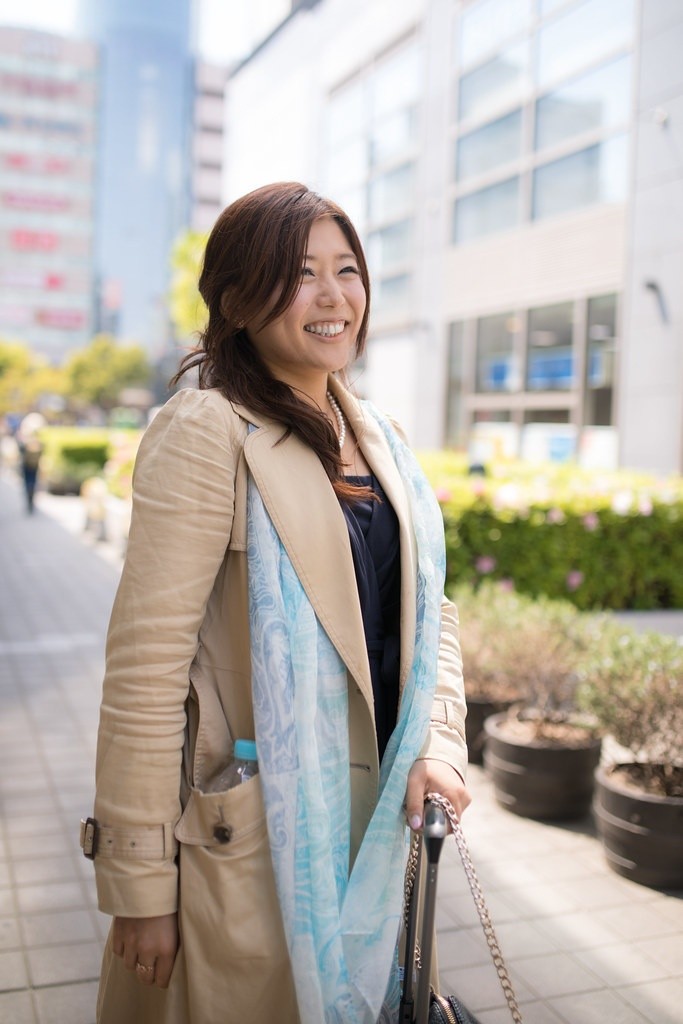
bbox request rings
[136,961,155,973]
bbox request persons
[19,430,45,512]
[77,183,472,1024]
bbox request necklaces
[326,391,351,449]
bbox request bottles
[206,739,259,794]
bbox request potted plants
[447,573,683,893]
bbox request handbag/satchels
[403,793,521,1024]
